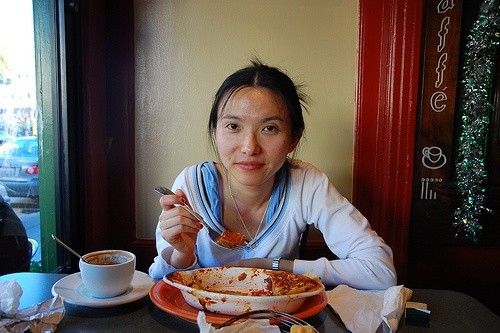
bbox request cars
[0,129,39,208]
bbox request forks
[153,185,245,253]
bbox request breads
[289,324,316,333]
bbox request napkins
[325,284,432,333]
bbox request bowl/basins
[163,265,326,316]
[79,250,138,299]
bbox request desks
[0,272,499,333]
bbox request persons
[0,195,32,276]
[150,48,397,291]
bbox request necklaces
[227,170,272,241]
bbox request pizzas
[218,230,245,247]
[270,271,318,296]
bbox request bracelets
[272,256,282,270]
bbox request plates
[51,271,154,307]
[150,278,328,327]
